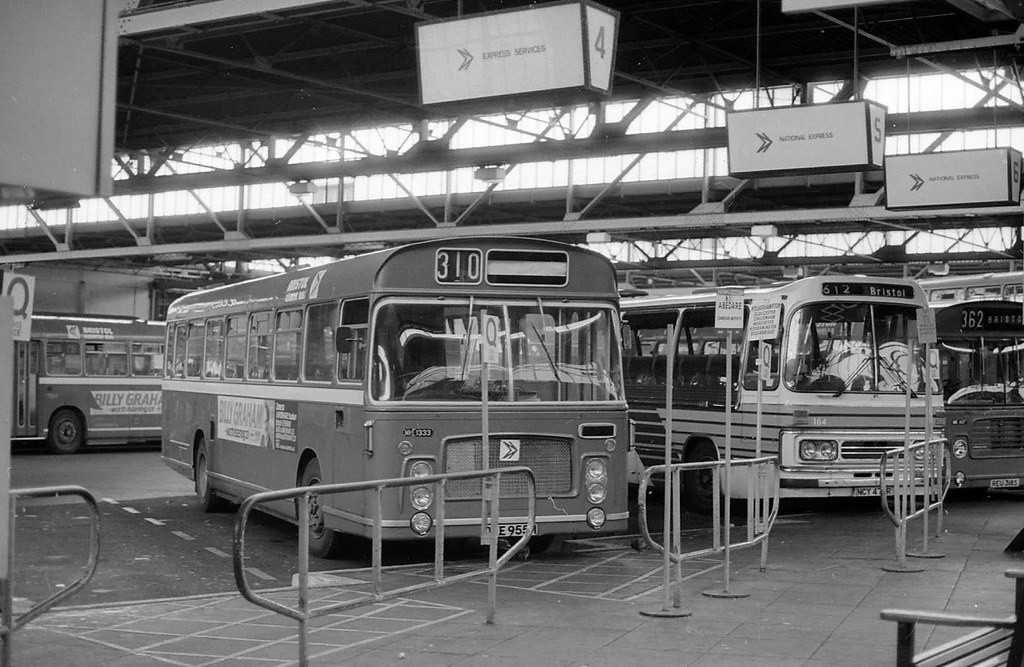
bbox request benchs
[881,570,1024,667]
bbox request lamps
[474,163,505,183]
[782,266,805,278]
[584,233,613,244]
[928,263,950,275]
[290,180,315,193]
[751,224,778,237]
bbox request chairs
[623,353,737,389]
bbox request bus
[160,235,633,560]
[852,298,1024,498]
[917,269,1024,303]
[13,315,174,453]
[611,273,946,513]
